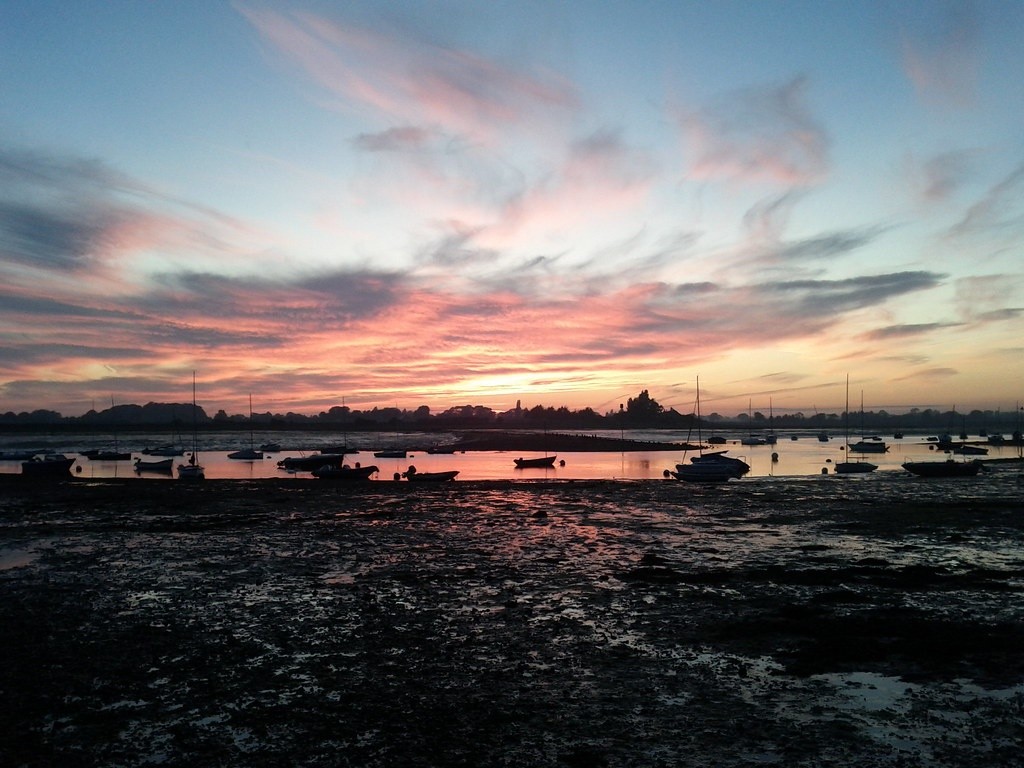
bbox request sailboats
[959,414,968,439]
[88,394,131,460]
[320,397,358,454]
[676,377,750,479]
[227,394,263,459]
[767,398,777,443]
[176,371,205,478]
[150,405,185,456]
[78,398,99,456]
[834,381,880,473]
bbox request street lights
[620,404,623,440]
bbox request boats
[311,466,379,479]
[791,435,798,440]
[954,445,989,455]
[987,435,1005,441]
[284,455,344,471]
[403,471,460,482]
[513,457,556,466]
[374,449,406,458]
[901,460,979,477]
[427,447,457,454]
[819,436,828,441]
[1012,431,1023,440]
[894,433,903,439]
[133,458,174,470]
[34,449,55,454]
[740,437,767,445]
[980,429,986,436]
[260,444,280,451]
[708,436,727,444]
[818,434,827,438]
[873,437,882,441]
[927,437,938,441]
[669,471,731,482]
[847,441,886,454]
